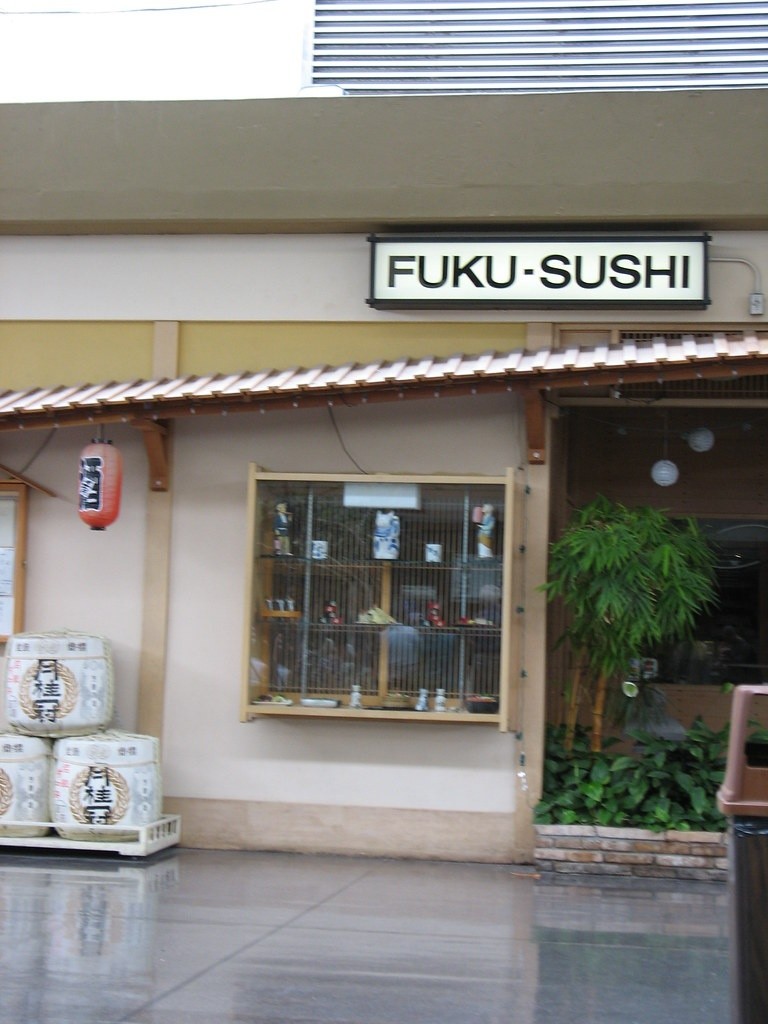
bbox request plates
[252,701,293,706]
[355,621,403,625]
[452,624,495,628]
[301,699,338,707]
[369,706,416,711]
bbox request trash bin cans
[716,685,768,1023]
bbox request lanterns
[76,439,123,531]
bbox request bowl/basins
[465,696,499,713]
[385,696,410,707]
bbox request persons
[273,504,294,555]
[378,621,425,696]
[477,504,496,558]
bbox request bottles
[435,689,446,712]
[416,689,428,711]
[349,685,362,710]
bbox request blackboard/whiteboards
[0,478,28,643]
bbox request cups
[312,541,328,560]
[426,544,442,562]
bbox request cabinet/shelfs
[244,463,522,733]
